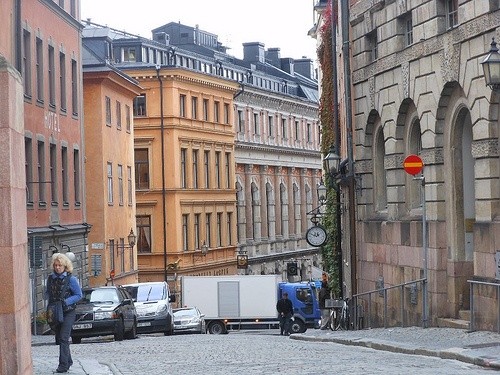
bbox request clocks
[307,226,326,247]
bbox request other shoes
[56,359,73,372]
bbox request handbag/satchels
[46,300,63,324]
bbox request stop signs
[404,153,424,176]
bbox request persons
[44,253,82,372]
[276,293,293,336]
[319,280,330,329]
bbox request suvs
[122,281,174,337]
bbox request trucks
[181,275,323,336]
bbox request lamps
[194,241,208,256]
[50,245,61,261]
[106,228,137,248]
[62,244,75,261]
[318,176,341,207]
[480,38,500,90]
[324,146,348,193]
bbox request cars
[172,307,207,335]
[70,284,138,344]
